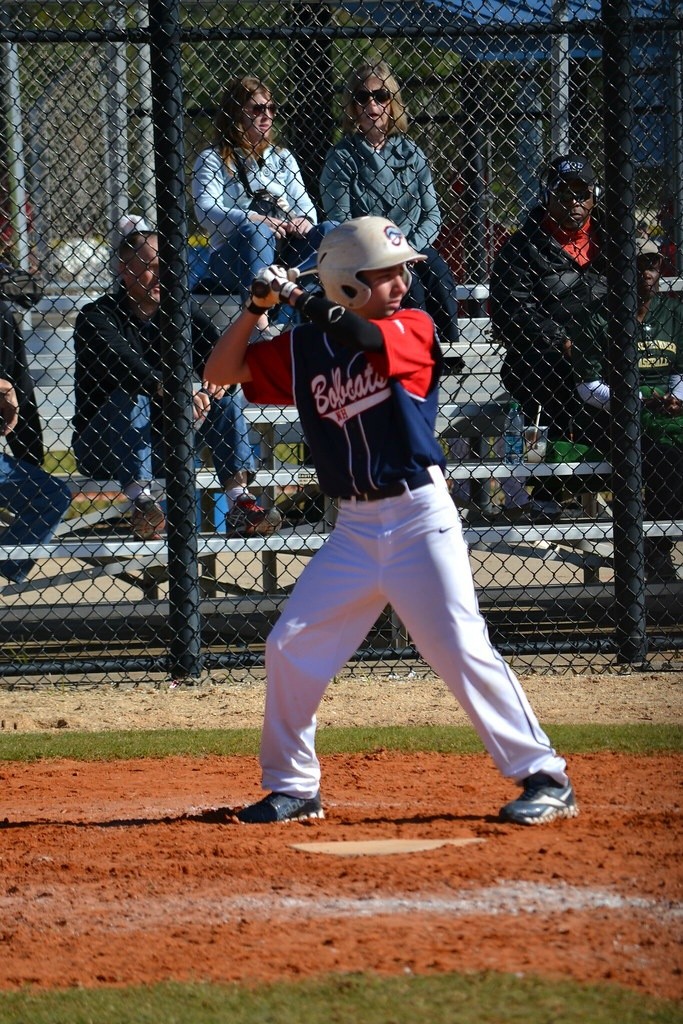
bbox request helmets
[317,215,428,310]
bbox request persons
[202,215,582,826]
[71,231,283,542]
[190,78,341,337]
[490,155,683,581]
[0,263,73,594]
[321,60,467,375]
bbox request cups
[522,426,550,462]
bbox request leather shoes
[339,469,434,501]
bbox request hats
[635,238,665,260]
[548,155,594,189]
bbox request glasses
[355,89,392,104]
[242,104,278,116]
[553,184,591,203]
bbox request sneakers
[499,772,580,826]
[232,791,325,824]
[131,496,166,541]
[225,492,282,537]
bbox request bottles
[504,401,524,465]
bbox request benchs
[0,276,683,646]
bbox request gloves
[244,263,300,316]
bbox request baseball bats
[252,268,319,299]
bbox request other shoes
[250,329,273,344]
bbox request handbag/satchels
[249,188,296,258]
